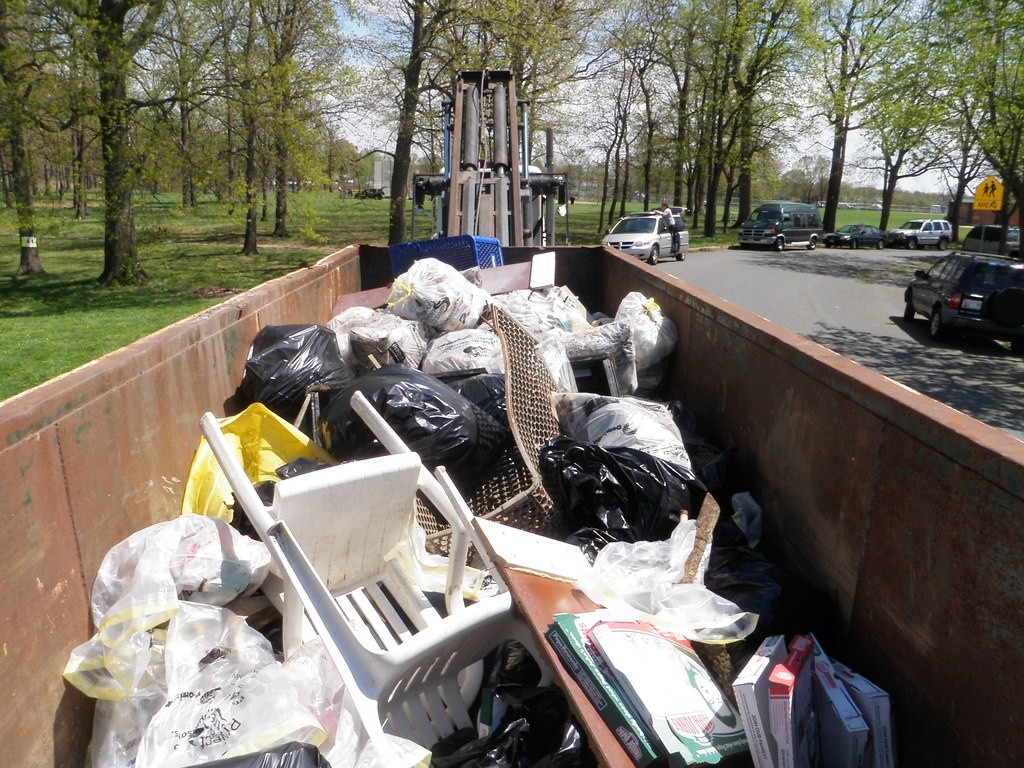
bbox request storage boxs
[546,605,893,768]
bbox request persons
[569,195,576,204]
[654,196,680,252]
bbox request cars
[822,223,894,249]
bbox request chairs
[201,304,570,768]
[968,271,1014,293]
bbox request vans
[737,201,824,251]
[602,207,690,266]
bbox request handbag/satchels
[62,516,272,702]
[133,600,328,768]
[574,519,759,645]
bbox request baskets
[389,235,504,278]
[413,300,569,564]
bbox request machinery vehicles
[406,68,573,246]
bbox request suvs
[886,219,954,251]
[904,250,1024,355]
[355,188,386,200]
[962,225,1021,258]
